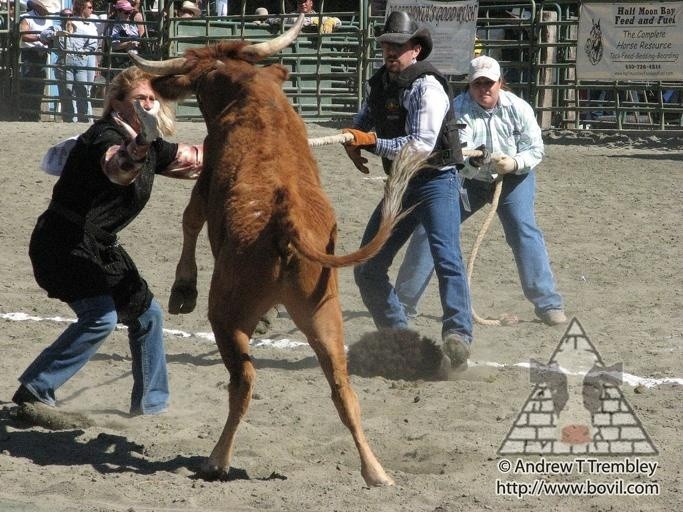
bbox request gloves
[469,144,491,166]
[133,98,161,141]
[495,154,516,175]
[345,144,370,175]
[340,128,378,149]
[311,18,336,36]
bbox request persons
[496,7,540,118]
[10,63,204,420]
[340,10,474,373]
[395,54,567,327]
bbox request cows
[126,12,438,488]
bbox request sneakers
[443,333,471,375]
[540,308,568,325]
[10,386,44,406]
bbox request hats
[469,55,501,82]
[112,0,135,11]
[372,11,433,62]
[31,0,73,16]
[505,8,531,21]
[179,0,202,16]
